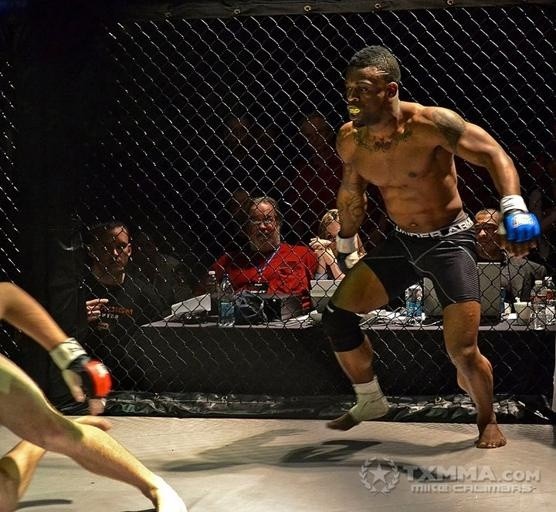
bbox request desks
[126,299,556,394]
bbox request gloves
[500,195,540,244]
[335,233,359,275]
[51,338,112,404]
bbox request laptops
[421,261,502,323]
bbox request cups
[513,302,534,320]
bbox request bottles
[206,270,220,316]
[217,272,236,328]
[544,276,556,327]
[530,280,547,331]
[404,282,423,327]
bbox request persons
[319,46,542,449]
[83,220,172,392]
[0,281,186,512]
[192,115,555,313]
